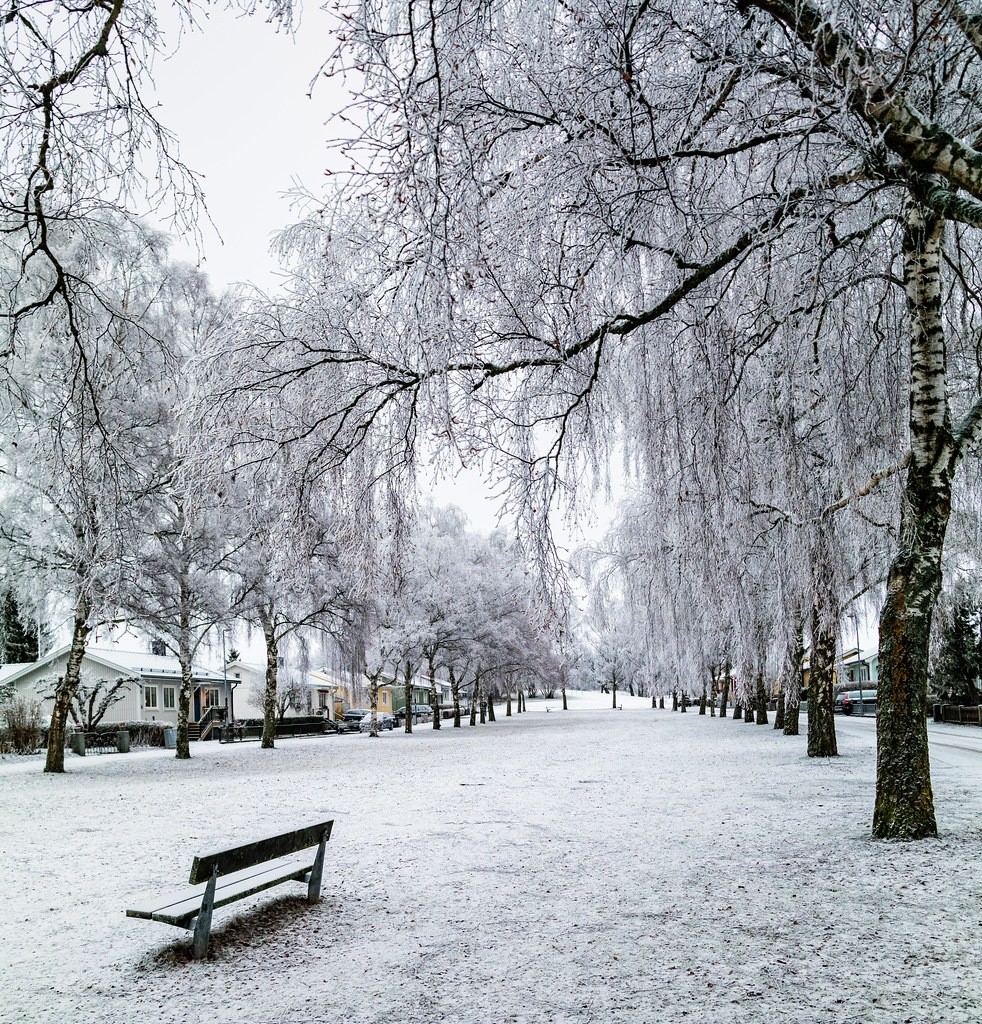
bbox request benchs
[125,819,334,959]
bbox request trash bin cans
[164,729,177,749]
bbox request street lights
[222,629,232,743]
[847,613,864,717]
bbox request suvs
[835,689,878,714]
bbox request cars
[393,704,434,720]
[337,708,394,734]
